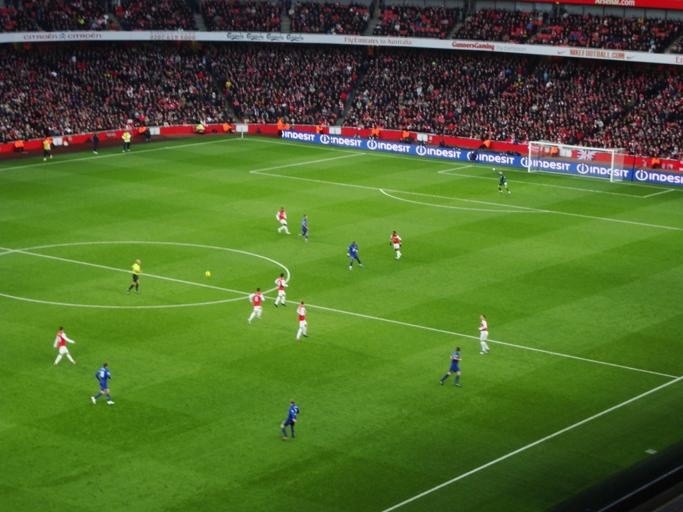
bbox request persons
[299,213,310,241]
[126,259,142,294]
[276,207,291,235]
[390,229,403,259]
[296,301,308,340]
[90,362,116,406]
[439,346,464,388]
[274,273,289,308]
[347,240,363,271]
[279,400,299,440]
[478,314,490,355]
[248,288,266,325]
[52,327,77,366]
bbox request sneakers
[91,397,115,406]
[347,253,403,272]
[498,190,511,195]
[282,435,297,440]
[127,290,141,296]
[246,301,285,326]
[53,360,78,367]
[296,334,307,341]
[480,348,490,355]
[439,379,462,390]
[277,228,309,242]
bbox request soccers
[205,271,210,276]
